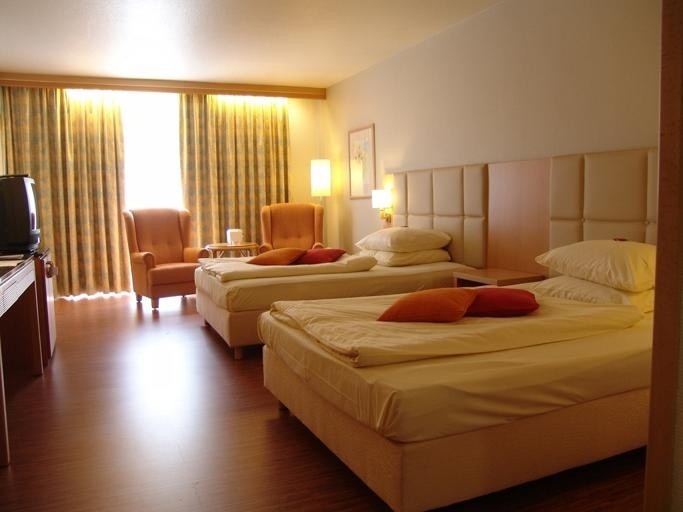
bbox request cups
[227,229,242,245]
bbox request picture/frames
[348,123,376,198]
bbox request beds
[257,284,655,512]
[194,253,475,363]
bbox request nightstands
[450,267,545,287]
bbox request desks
[204,241,255,258]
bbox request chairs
[123,207,203,310]
[259,199,324,253]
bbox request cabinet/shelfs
[0,249,59,467]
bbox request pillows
[250,248,305,264]
[470,289,538,317]
[354,227,452,250]
[300,247,346,263]
[534,237,656,291]
[377,286,475,320]
[366,251,452,267]
[528,276,655,307]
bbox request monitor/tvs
[0,176,42,254]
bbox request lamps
[310,160,332,205]
[371,190,392,225]
